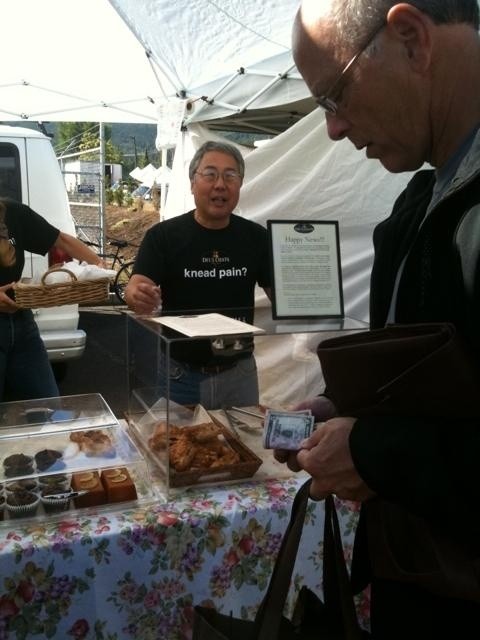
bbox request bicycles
[80,233,137,306]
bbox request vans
[76,182,95,198]
[0,124,77,284]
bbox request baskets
[13,263,112,310]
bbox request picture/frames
[267,218,344,319]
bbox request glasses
[313,22,385,117]
[195,169,243,184]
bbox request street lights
[130,135,137,169]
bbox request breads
[0,430,137,521]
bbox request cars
[110,181,161,208]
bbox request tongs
[222,403,266,438]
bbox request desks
[0,406,372,640]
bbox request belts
[171,354,252,377]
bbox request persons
[122,140,273,412]
[1,201,106,426]
[272,0,478,638]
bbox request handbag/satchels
[189,479,379,638]
[315,320,479,598]
